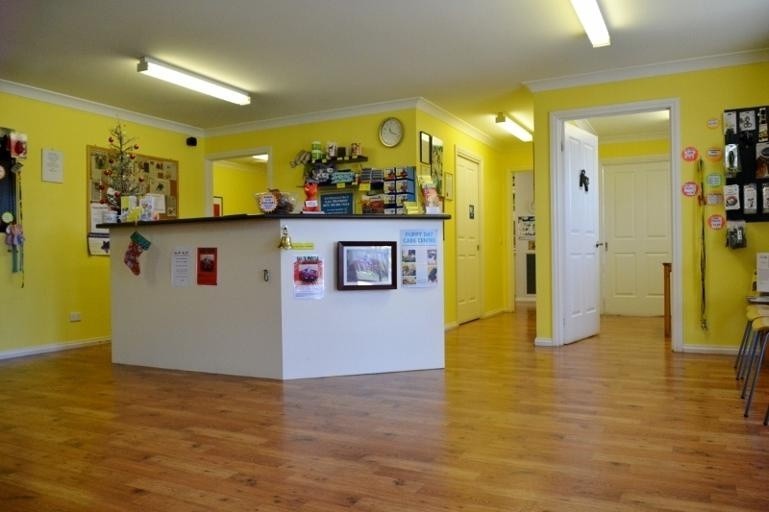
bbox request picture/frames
[419,131,432,166]
[336,240,398,292]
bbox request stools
[732,296,769,426]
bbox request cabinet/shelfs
[295,155,383,190]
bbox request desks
[92,211,450,382]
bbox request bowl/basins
[254,192,299,214]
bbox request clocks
[377,115,403,148]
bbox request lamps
[136,55,252,106]
[496,112,533,144]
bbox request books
[416,174,441,214]
